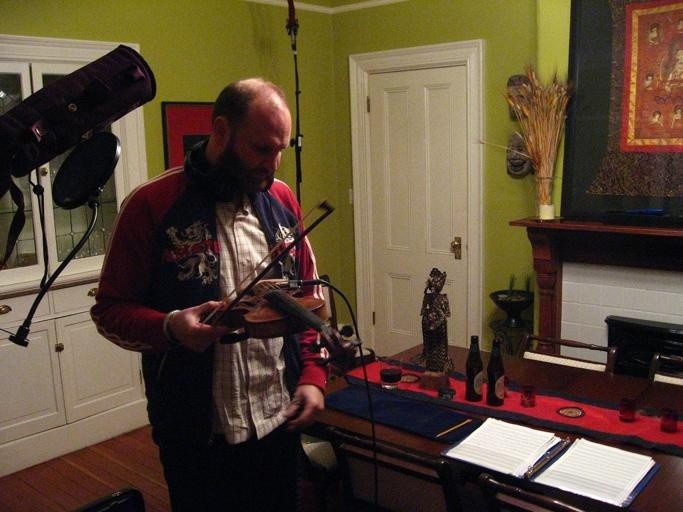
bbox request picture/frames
[162,102,215,169]
[561,0,683,227]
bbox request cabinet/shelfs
[1,34,147,475]
[509,218,683,432]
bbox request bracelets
[164,310,181,348]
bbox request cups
[378,354,403,390]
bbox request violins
[88,201,362,370]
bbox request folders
[441,417,659,507]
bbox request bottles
[486,339,504,406]
[465,335,482,402]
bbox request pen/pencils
[436,419,472,437]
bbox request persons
[91,77,332,511]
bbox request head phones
[183,137,275,203]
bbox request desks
[296,342,683,512]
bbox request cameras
[1,44,158,348]
